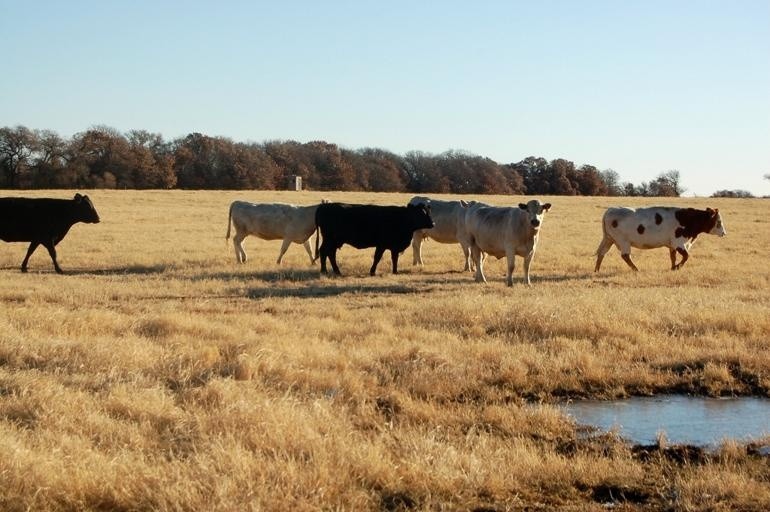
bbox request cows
[224,199,332,267]
[463,199,553,287]
[407,195,482,274]
[312,200,435,275]
[589,205,730,274]
[0,192,102,274]
